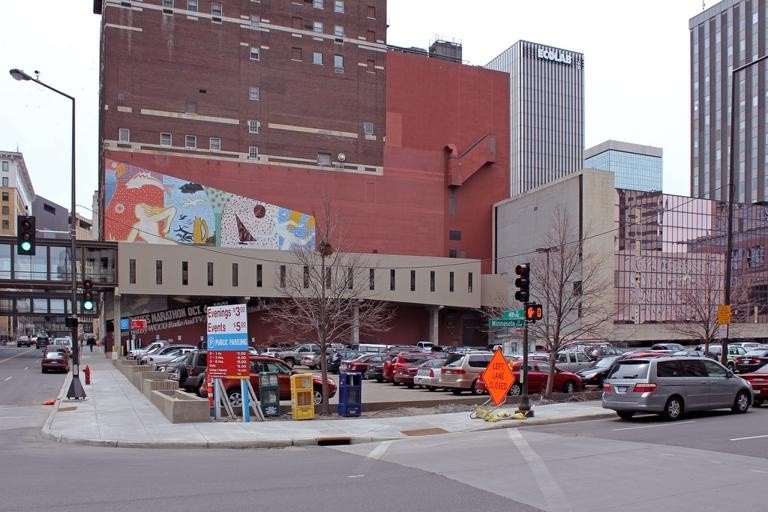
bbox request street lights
[9,69,86,401]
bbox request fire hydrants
[81,364,90,385]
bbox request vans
[601,356,753,421]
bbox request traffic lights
[515,263,529,301]
[16,214,35,254]
[526,304,543,320]
[84,280,94,312]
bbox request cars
[41,352,69,373]
[16,335,73,357]
[43,344,68,353]
[695,341,768,374]
[622,352,671,359]
[651,344,685,352]
[198,355,336,408]
[672,350,719,364]
[739,364,768,408]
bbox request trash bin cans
[289,372,316,421]
[259,371,281,417]
[338,370,363,418]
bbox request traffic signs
[489,320,524,329]
[503,309,524,319]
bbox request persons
[87,335,97,351]
